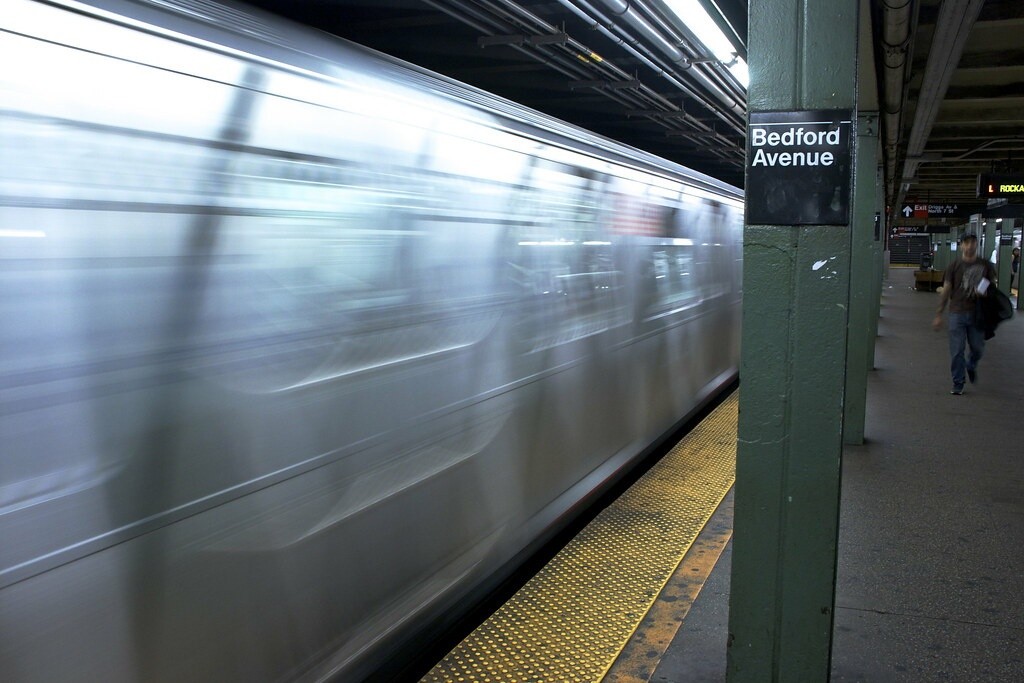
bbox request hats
[960,232,977,242]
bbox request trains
[0,2,747,682]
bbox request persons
[1010,247,1019,297]
[932,233,996,396]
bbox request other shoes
[1009,293,1015,296]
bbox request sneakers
[950,383,965,395]
[967,366,978,385]
